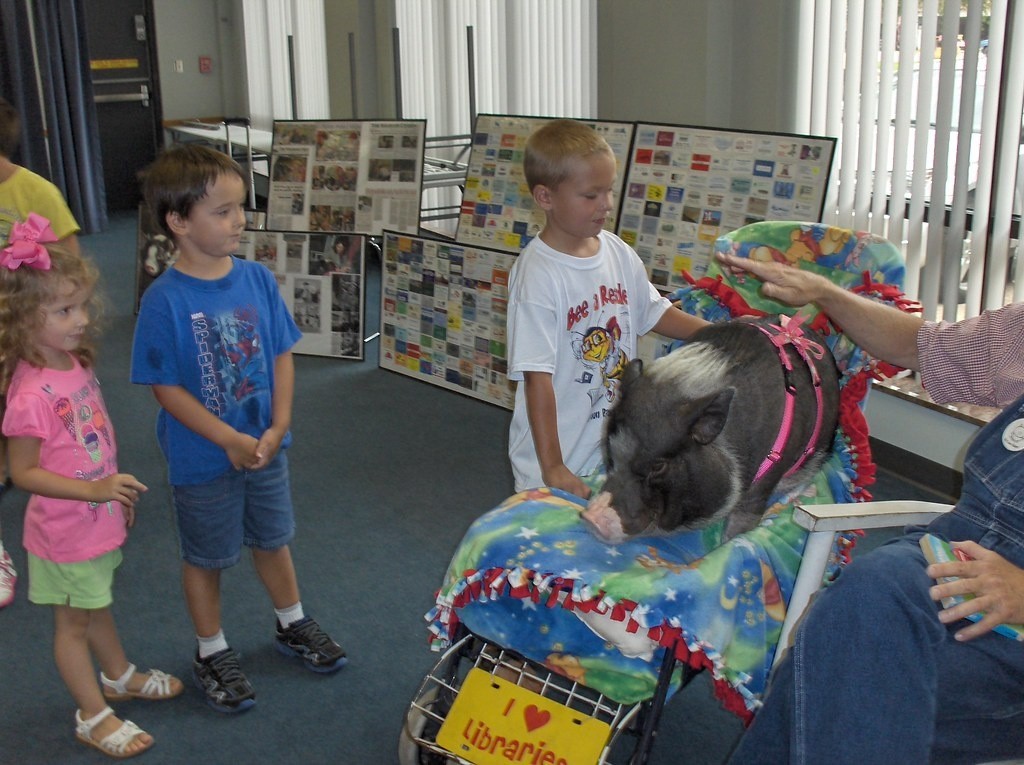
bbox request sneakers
[191,640,257,716]
[0,550,18,606]
[275,611,348,671]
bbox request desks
[167,25,481,223]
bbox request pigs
[580,313,841,544]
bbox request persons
[0,213,185,757]
[716,251,1024,765]
[130,143,350,712]
[331,235,352,273]
[506,119,763,499]
[280,126,305,146]
[0,96,80,606]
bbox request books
[919,533,1024,641]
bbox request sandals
[75,704,155,758]
[100,661,184,701]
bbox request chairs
[770,482,954,693]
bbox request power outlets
[174,60,184,74]
